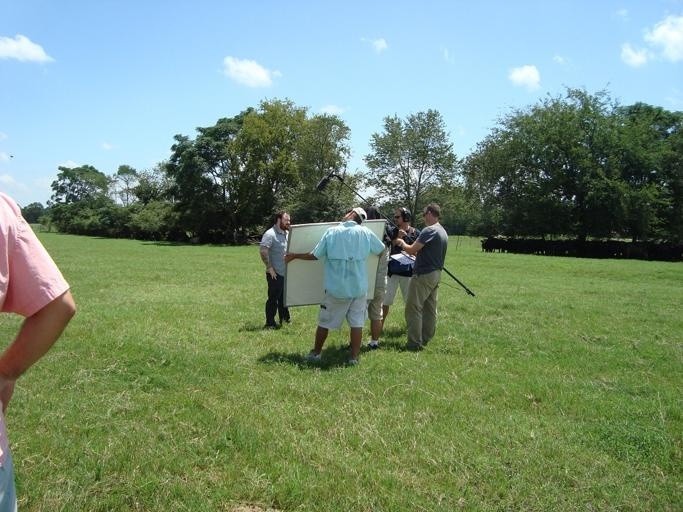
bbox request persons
[258,208,292,330]
[359,203,393,351]
[282,206,386,365]
[381,207,421,333]
[0,191,76,512]
[391,203,448,352]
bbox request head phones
[402,207,410,222]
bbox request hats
[353,207,367,223]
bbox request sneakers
[366,344,380,350]
[308,350,324,361]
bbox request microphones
[317,176,331,191]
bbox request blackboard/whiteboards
[281,218,389,309]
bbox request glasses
[395,215,400,219]
